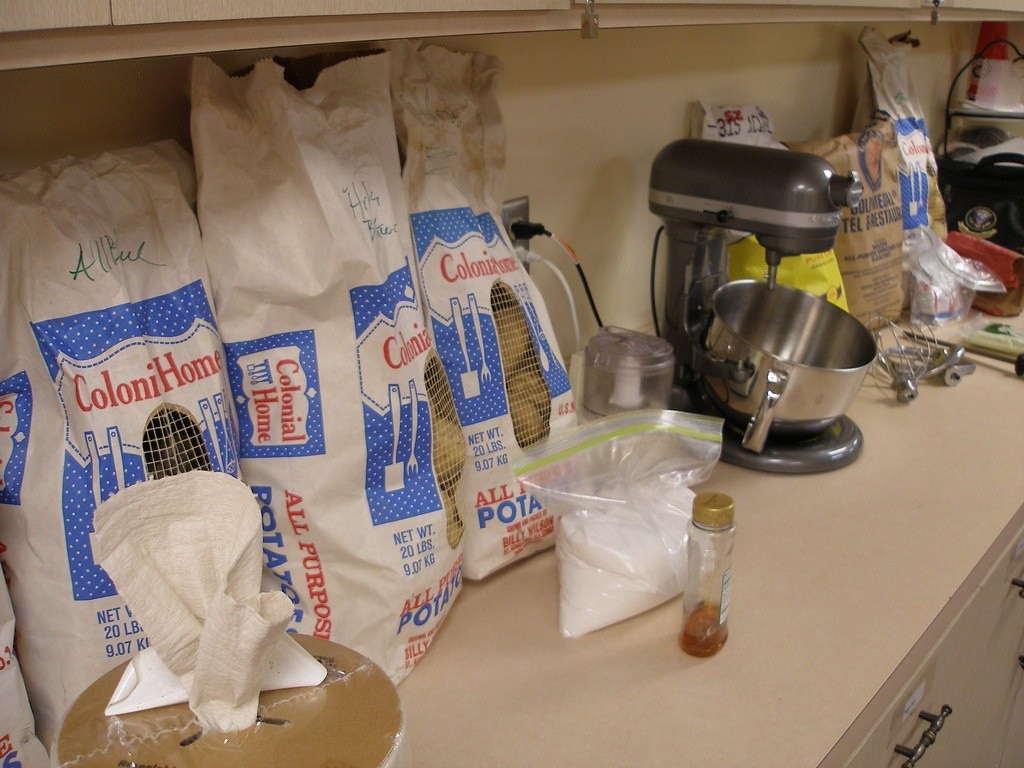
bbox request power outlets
[502,193,532,277]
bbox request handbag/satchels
[935,152,1024,258]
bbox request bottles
[679,491,737,656]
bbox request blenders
[647,137,882,476]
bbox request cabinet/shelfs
[833,534,1024,767]
